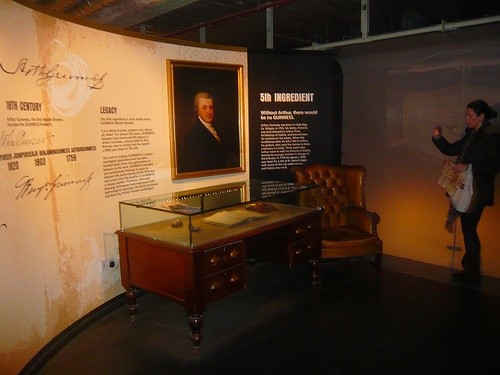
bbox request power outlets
[102,258,116,272]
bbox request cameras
[434,129,440,138]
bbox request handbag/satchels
[451,164,473,213]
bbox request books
[201,208,269,228]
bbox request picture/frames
[166,59,247,180]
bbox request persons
[187,93,225,173]
[432,100,500,286]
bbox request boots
[452,254,480,285]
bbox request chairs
[292,163,383,272]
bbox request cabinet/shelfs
[116,201,324,354]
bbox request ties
[210,127,222,142]
